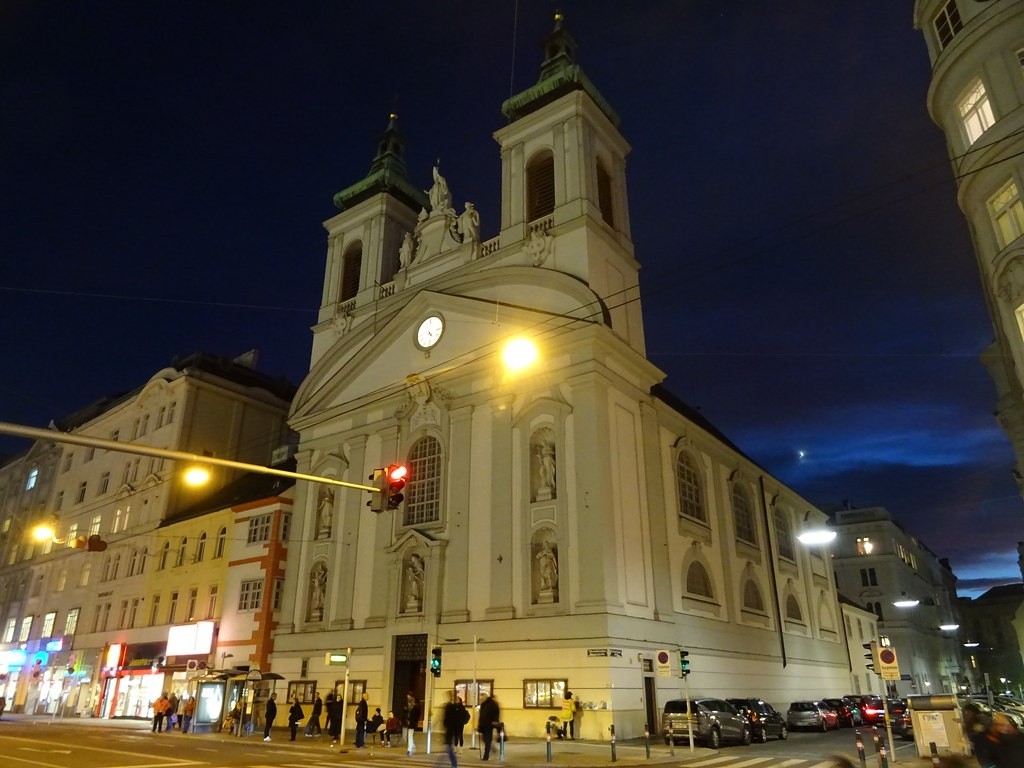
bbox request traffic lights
[68,655,78,674]
[30,659,42,679]
[388,464,408,511]
[862,643,874,671]
[431,642,444,677]
[679,650,690,681]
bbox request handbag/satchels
[171,714,178,723]
[464,710,471,724]
[227,711,233,718]
[164,708,173,716]
[496,721,508,743]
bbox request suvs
[822,697,864,728]
[726,697,788,744]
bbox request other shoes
[151,729,155,733]
[483,757,488,761]
[264,736,273,742]
[313,733,322,738]
[333,739,337,744]
[359,745,367,749]
[157,730,163,733]
[412,746,416,753]
[459,747,464,754]
[288,738,296,742]
[305,733,313,737]
[381,741,384,746]
[569,737,575,740]
[562,736,568,740]
[228,732,230,735]
[453,745,458,753]
[407,751,412,756]
[182,731,187,734]
[384,742,391,748]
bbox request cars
[788,700,841,734]
[661,697,752,749]
[844,693,1024,746]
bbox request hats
[162,692,169,700]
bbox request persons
[263,692,277,742]
[456,202,481,242]
[305,691,322,738]
[537,441,556,488]
[535,540,558,590]
[424,167,448,209]
[477,695,500,760]
[407,555,424,600]
[439,691,471,768]
[354,693,383,750]
[223,696,255,737]
[561,692,576,740]
[311,564,327,609]
[402,692,422,756]
[149,692,196,734]
[288,698,304,741]
[380,711,401,748]
[325,690,343,744]
[399,231,414,269]
[320,486,332,529]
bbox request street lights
[470,633,486,752]
[219,651,234,671]
